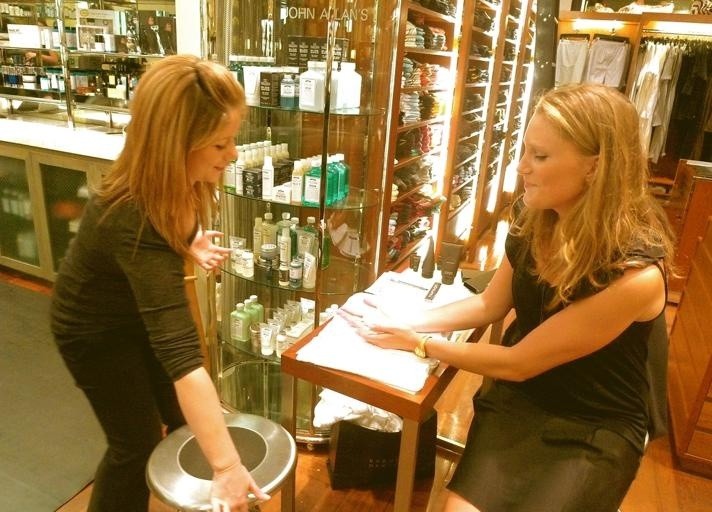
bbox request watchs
[414,336,432,360]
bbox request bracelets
[212,459,241,477]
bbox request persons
[356,81,680,511]
[46,49,273,512]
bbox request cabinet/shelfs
[665,158,712,479]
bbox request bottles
[223,139,352,358]
[0,0,137,101]
[1,189,32,219]
[279,59,363,116]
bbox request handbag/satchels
[326,408,437,489]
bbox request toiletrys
[222,294,349,359]
[217,210,339,290]
[216,137,352,207]
[218,58,366,118]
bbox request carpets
[1,278,110,510]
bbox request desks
[281,269,505,511]
